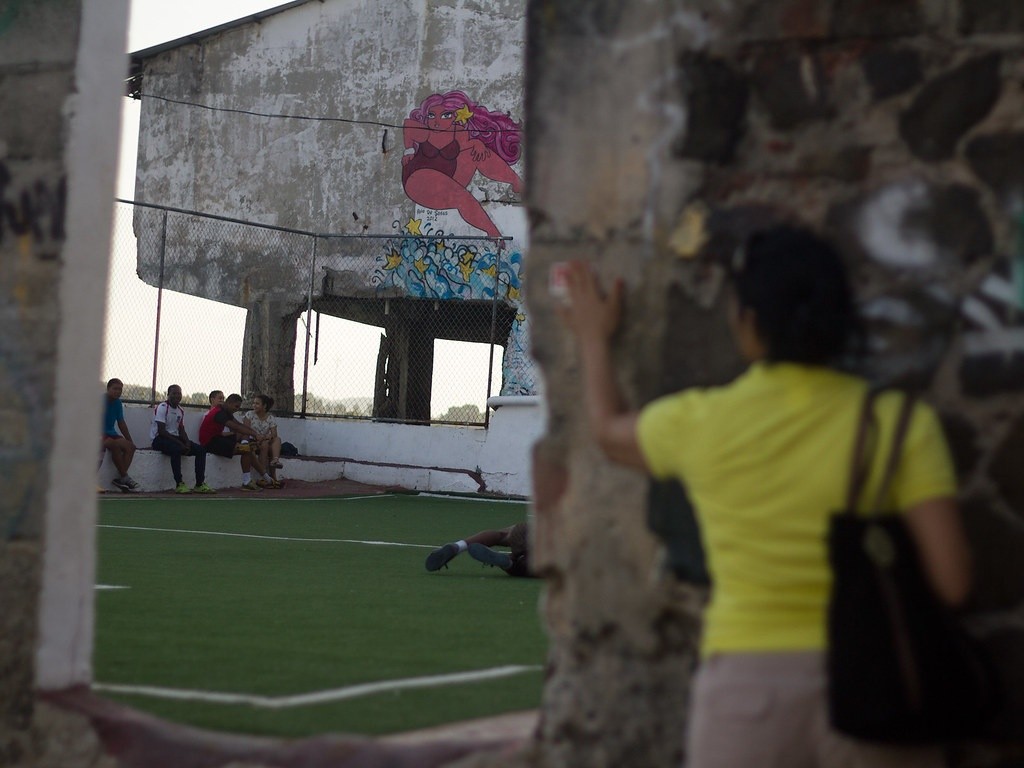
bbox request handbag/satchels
[280,442,299,457]
[824,378,1024,750]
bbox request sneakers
[240,479,263,492]
[112,477,143,492]
[193,481,216,494]
[175,482,192,494]
[236,442,261,453]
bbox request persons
[199,389,283,492]
[549,221,984,768]
[424,521,528,576]
[150,384,216,493]
[101,377,141,492]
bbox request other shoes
[466,542,512,570]
[426,542,458,572]
[271,479,281,489]
[96,484,109,493]
[257,480,274,489]
[269,458,283,469]
